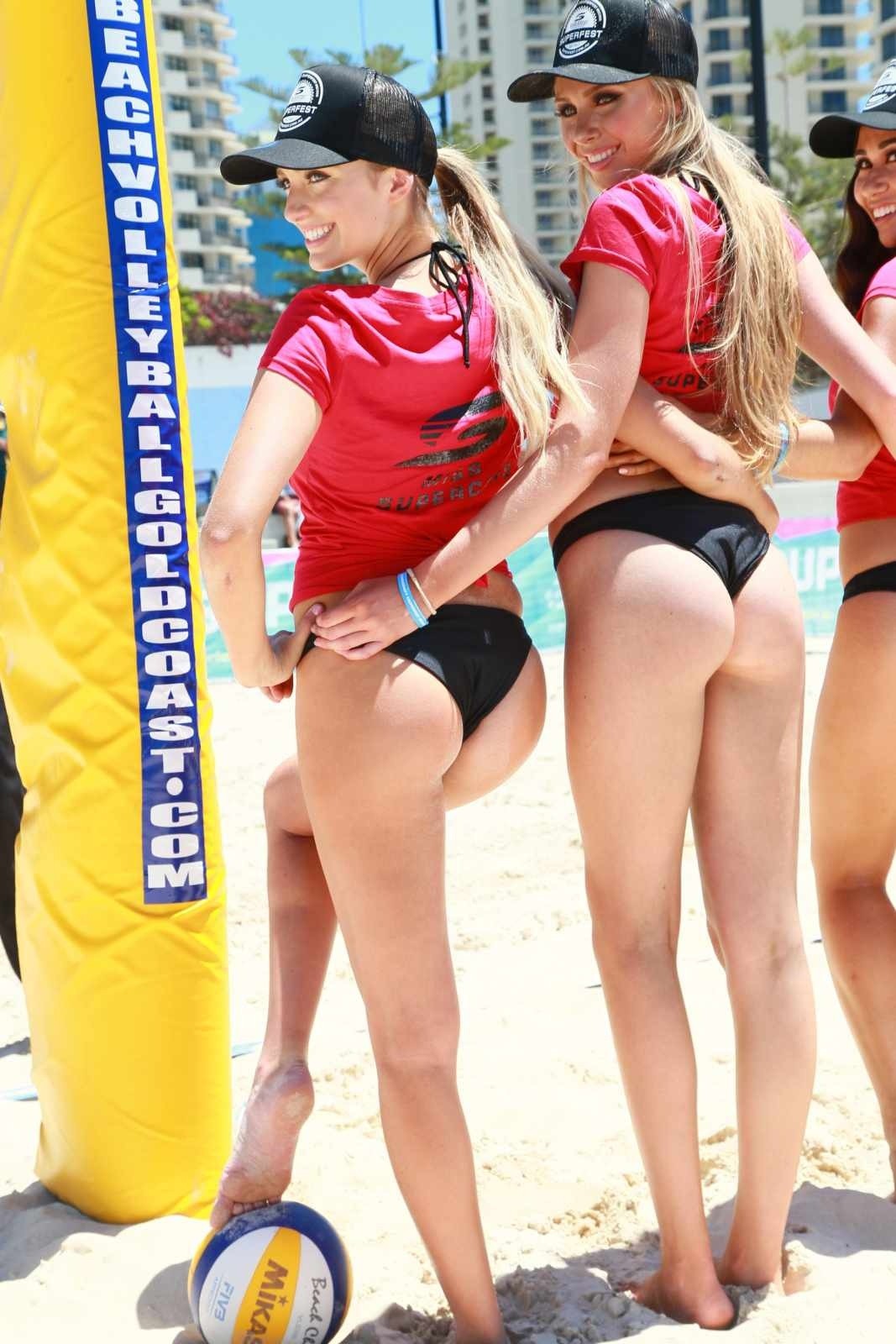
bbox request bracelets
[772,417,791,476]
[396,571,429,628]
[406,567,437,616]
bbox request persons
[173,0,896,1344]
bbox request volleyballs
[188,1201,354,1344]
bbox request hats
[506,1,698,102]
[809,60,895,159]
[220,66,437,186]
